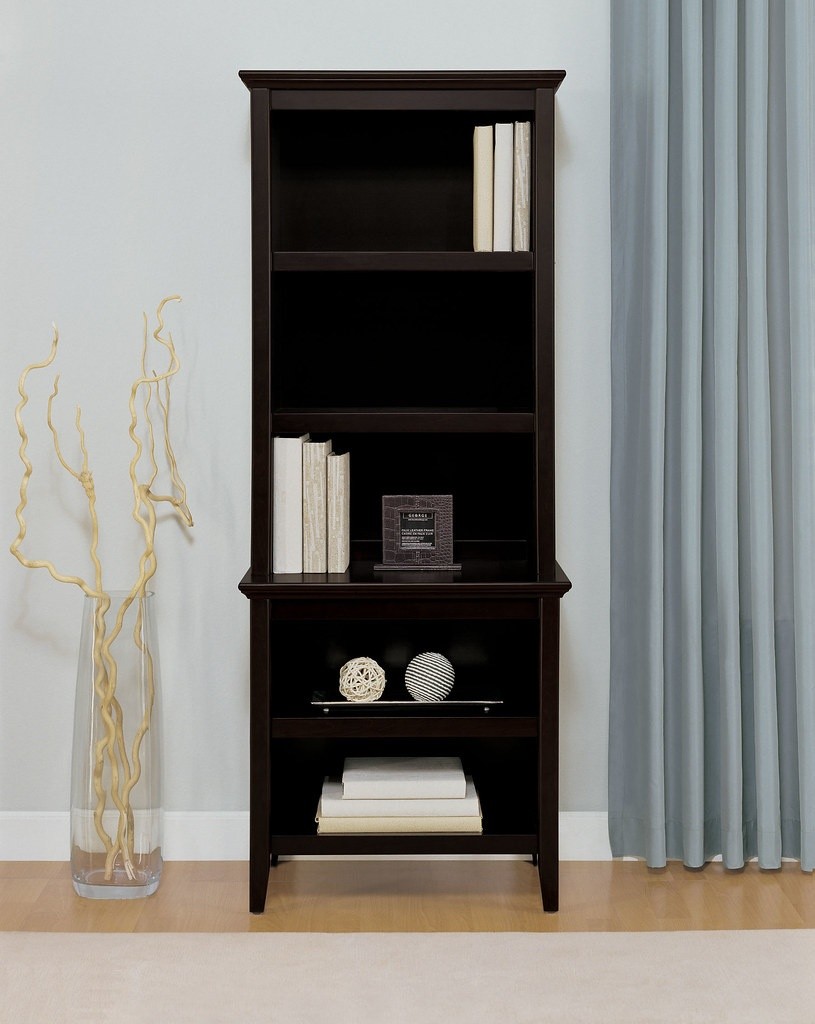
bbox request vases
[70,588,163,899]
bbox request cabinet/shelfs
[238,68,572,914]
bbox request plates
[310,689,504,707]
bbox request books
[472,122,533,253]
[317,755,483,834]
[273,434,349,574]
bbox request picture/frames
[381,494,454,566]
